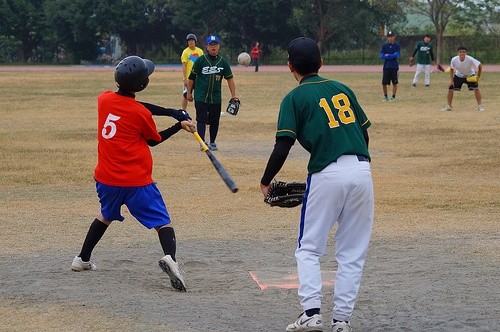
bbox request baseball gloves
[226,98,240,115]
[263,179,306,209]
[408,58,415,67]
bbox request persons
[250,42,262,73]
[260,37,374,332]
[380,31,400,103]
[180,34,203,110]
[441,46,485,113]
[411,35,436,87]
[186,36,238,152]
[69,55,196,291]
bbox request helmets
[114,56,155,92]
[186,33,197,44]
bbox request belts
[332,154,368,162]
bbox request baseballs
[238,52,251,65]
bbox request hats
[205,35,220,45]
[387,31,395,37]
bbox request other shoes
[478,104,484,111]
[441,105,452,112]
[382,96,389,102]
[389,97,395,101]
[200,142,218,151]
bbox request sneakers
[71,256,97,271]
[158,255,187,292]
[332,318,352,332]
[285,311,322,332]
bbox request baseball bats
[182,109,238,194]
